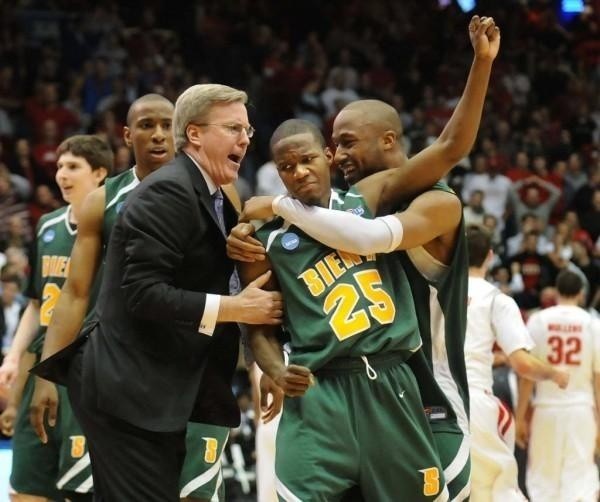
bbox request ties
[214,188,256,373]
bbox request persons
[2,135,111,501]
[235,15,506,501]
[0,0,205,347]
[456,223,567,498]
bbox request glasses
[190,122,255,138]
